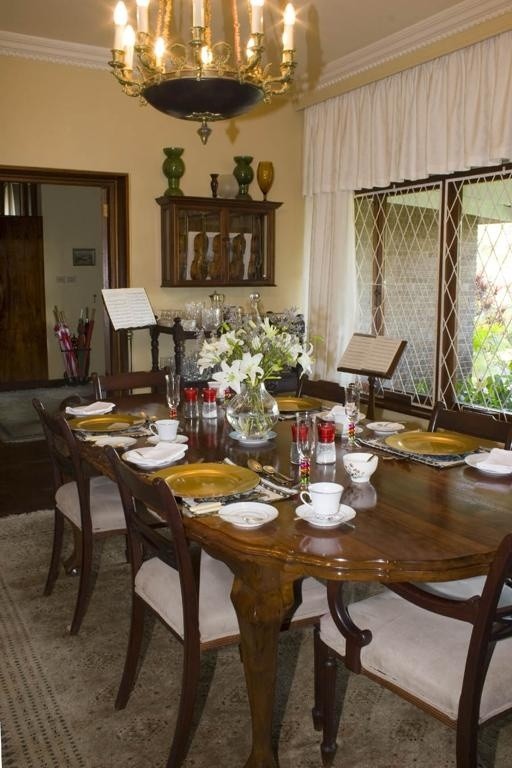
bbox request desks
[149,310,306,395]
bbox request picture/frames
[72,246,96,266]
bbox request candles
[125,46,133,67]
[193,0,204,27]
[282,24,294,50]
[251,4,265,33]
[136,5,150,34]
[113,25,124,50]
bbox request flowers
[197,317,322,392]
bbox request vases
[224,380,281,440]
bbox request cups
[160,304,246,329]
[300,483,344,515]
[150,420,179,440]
[163,355,213,380]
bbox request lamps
[108,0,296,144]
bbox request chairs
[31,394,169,636]
[316,530,512,767]
[428,399,512,448]
[90,364,173,400]
[296,373,357,405]
[102,442,330,766]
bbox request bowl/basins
[343,452,377,482]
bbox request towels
[66,401,115,415]
[326,404,346,420]
[130,441,189,464]
[478,448,512,474]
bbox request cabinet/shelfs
[155,194,283,287]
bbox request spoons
[247,459,287,484]
[263,465,293,482]
[210,512,262,521]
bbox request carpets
[1,382,107,442]
[2,507,498,764]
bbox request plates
[67,407,111,415]
[147,436,188,443]
[385,434,477,454]
[122,448,185,467]
[466,452,512,474]
[96,438,138,448]
[274,397,322,411]
[221,502,279,528]
[367,422,406,431]
[296,504,355,527]
[69,416,146,433]
[146,465,260,498]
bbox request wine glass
[166,375,180,419]
[345,381,360,446]
[296,413,317,492]
